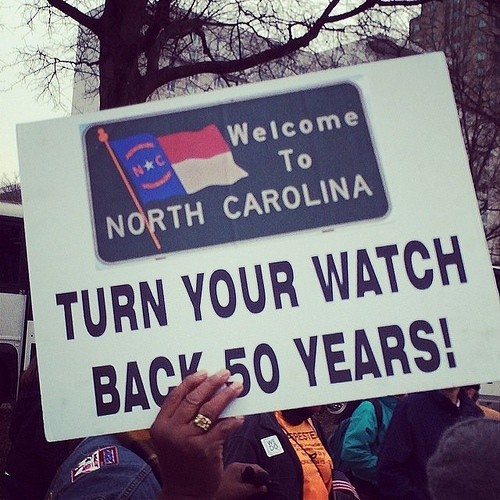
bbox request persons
[0,355,500,500]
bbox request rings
[194,413,212,431]
